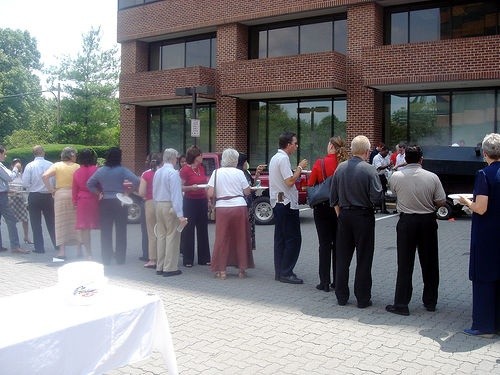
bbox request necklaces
[192,168,201,176]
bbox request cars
[126,174,142,224]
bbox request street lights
[172,86,214,144]
[296,106,329,152]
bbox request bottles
[177,218,188,232]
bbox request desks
[0,284,179,375]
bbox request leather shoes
[424,304,439,313]
[385,305,410,316]
[275,273,303,284]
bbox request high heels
[317,281,330,292]
[24,238,34,244]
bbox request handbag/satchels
[210,208,217,220]
[307,158,332,205]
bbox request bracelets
[298,166,303,170]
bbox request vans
[384,145,488,221]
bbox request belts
[343,206,367,209]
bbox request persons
[269,132,310,284]
[131,153,163,268]
[207,147,264,279]
[85,147,141,266]
[370,141,407,213]
[178,146,211,267]
[458,132,500,335]
[328,135,383,308]
[73,149,102,261]
[385,145,446,315]
[0,146,34,254]
[41,147,84,258]
[152,148,186,277]
[307,135,351,292]
[21,146,59,254]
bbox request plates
[250,187,268,189]
[116,193,133,204]
[448,194,473,200]
[197,184,208,187]
[154,224,157,237]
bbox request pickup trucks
[179,152,312,226]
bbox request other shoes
[12,247,29,254]
[359,299,372,309]
[144,261,157,268]
[56,255,64,259]
[239,271,247,279]
[215,271,227,280]
[205,261,210,264]
[463,328,496,338]
[381,210,389,214]
[139,256,148,262]
[32,249,38,254]
[156,271,163,274]
[163,270,181,277]
[186,263,192,267]
[337,300,346,304]
[0,247,7,252]
